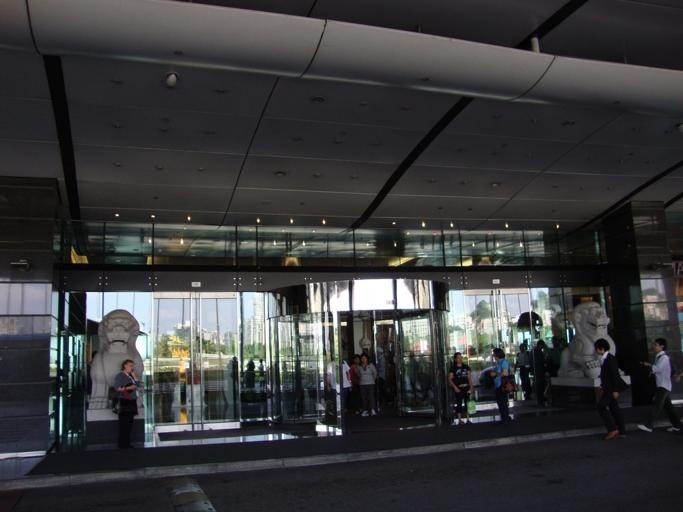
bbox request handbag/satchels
[500,374,519,394]
[466,400,478,416]
[108,386,136,401]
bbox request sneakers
[666,427,680,432]
[636,424,654,432]
[459,420,473,425]
[354,408,384,417]
[604,430,626,442]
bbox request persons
[447,351,473,426]
[404,350,433,406]
[489,347,513,426]
[227,356,266,390]
[85,350,97,412]
[114,359,139,448]
[594,338,628,443]
[327,345,387,417]
[514,335,566,401]
[637,338,682,433]
